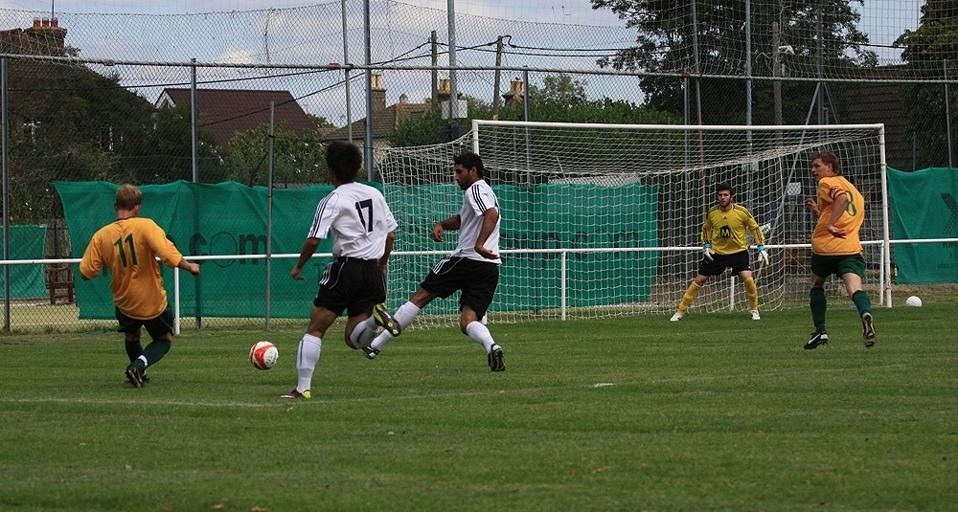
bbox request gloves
[758,246,769,266]
[703,244,716,264]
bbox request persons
[277,139,399,402]
[801,150,877,353]
[360,150,505,373]
[78,182,201,389]
[668,182,771,322]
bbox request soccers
[906,295,922,306]
[249,340,279,369]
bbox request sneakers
[804,330,829,350]
[488,344,507,372]
[862,312,876,348]
[280,387,311,399]
[373,303,401,337]
[670,313,683,321]
[750,309,760,320]
[125,364,150,389]
[362,344,380,360]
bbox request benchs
[45,258,75,305]
